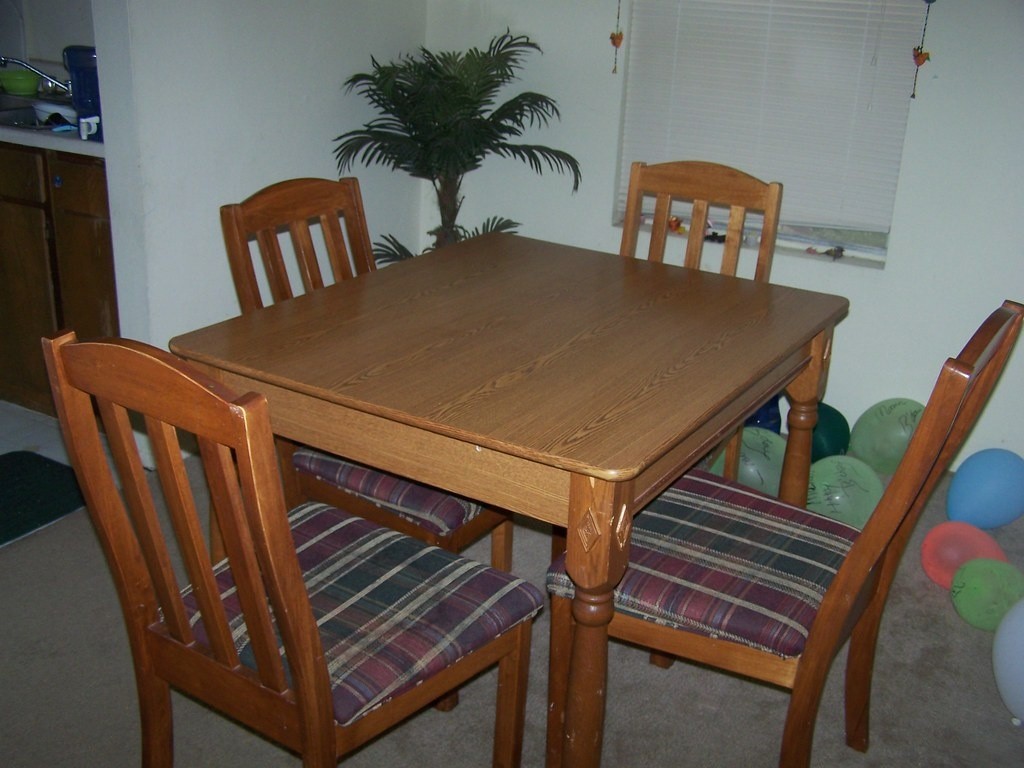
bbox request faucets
[0,56,73,98]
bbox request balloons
[916,448,1024,723]
[706,393,927,532]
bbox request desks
[170,232,847,766]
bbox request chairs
[218,177,516,575]
[621,160,782,480]
[543,301,1020,768]
[40,332,544,768]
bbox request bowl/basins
[0,68,42,94]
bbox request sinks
[0,103,49,132]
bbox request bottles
[64,45,101,128]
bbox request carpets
[0,452,86,546]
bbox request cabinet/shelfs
[1,143,129,427]
